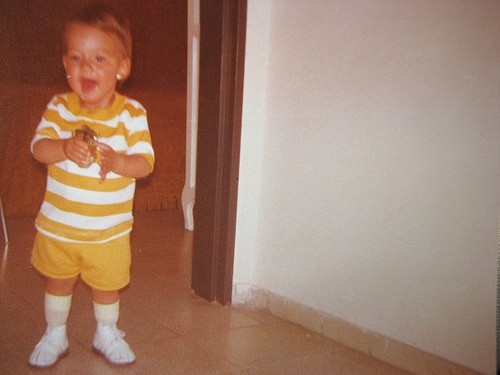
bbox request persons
[28,10,156,369]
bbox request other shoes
[92,322,137,367]
[30,330,70,367]
